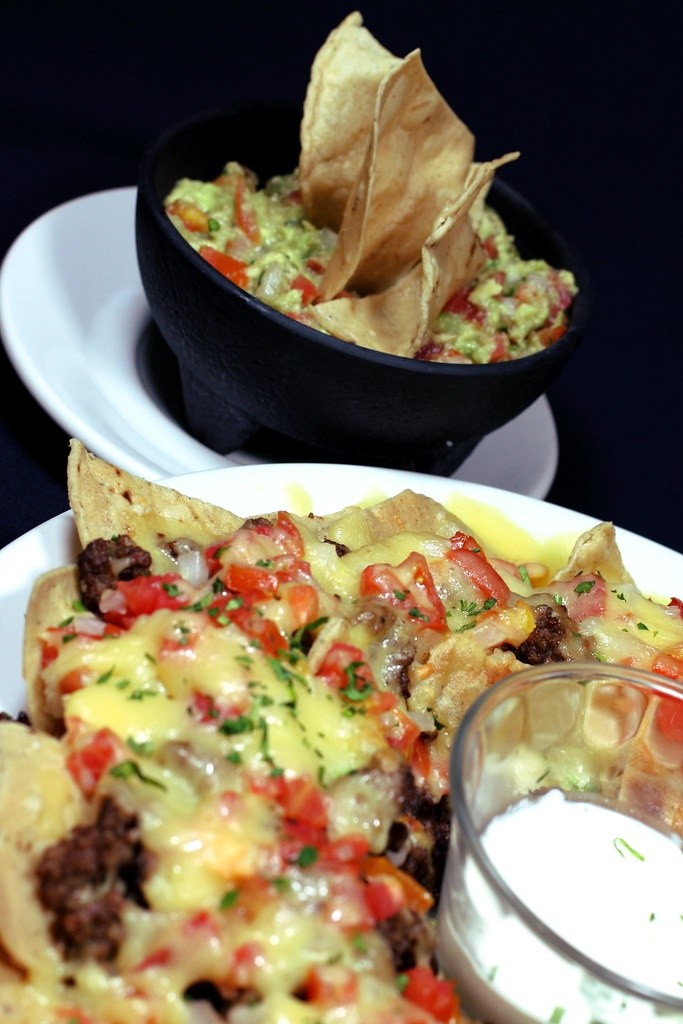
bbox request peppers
[172,174,568,363]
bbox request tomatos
[46,513,683,1024]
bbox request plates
[0,457,683,1024]
[1,182,562,519]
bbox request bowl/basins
[137,98,582,479]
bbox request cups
[431,652,682,1024]
